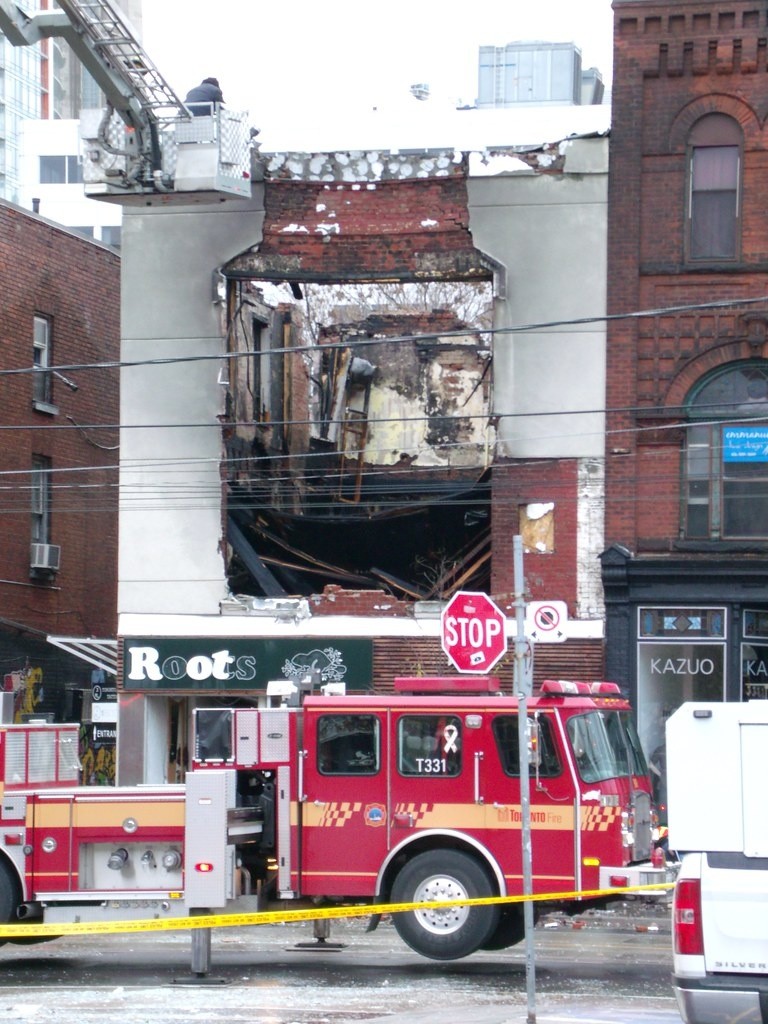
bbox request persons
[177,75,224,117]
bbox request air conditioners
[29,542,61,571]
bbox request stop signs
[440,589,508,676]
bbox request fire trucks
[1,670,677,959]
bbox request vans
[663,696,768,1023]
